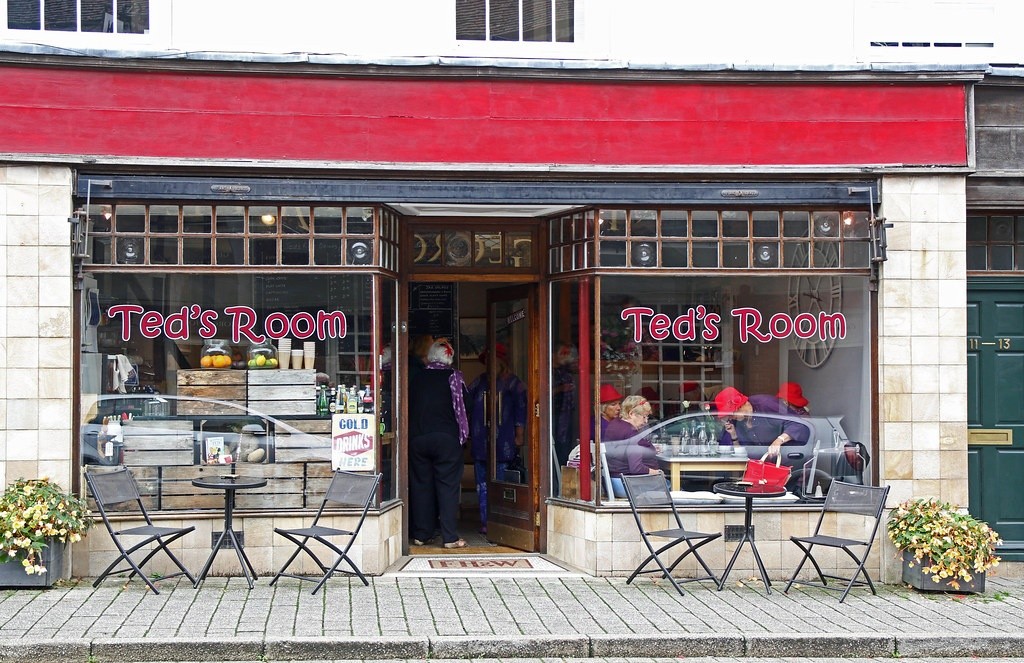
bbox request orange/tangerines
[201,355,231,368]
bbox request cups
[291,350,303,369]
[670,435,680,456]
[304,342,315,369]
[278,339,291,369]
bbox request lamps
[754,242,778,265]
[843,212,856,229]
[348,239,371,265]
[101,208,112,220]
[812,211,836,237]
[258,215,276,226]
[631,240,656,267]
[115,237,142,264]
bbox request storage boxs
[82,367,381,508]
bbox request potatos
[224,434,265,462]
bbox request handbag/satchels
[742,452,794,493]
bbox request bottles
[200,339,232,370]
[679,420,718,456]
[246,338,278,369]
[660,426,669,452]
[319,385,373,413]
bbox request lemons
[248,354,278,366]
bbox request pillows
[637,488,723,504]
[716,492,800,504]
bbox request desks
[712,482,787,596]
[655,454,750,491]
[190,473,270,590]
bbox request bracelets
[778,436,784,442]
[732,437,738,441]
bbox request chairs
[268,466,385,595]
[784,478,891,604]
[84,463,198,594]
[618,468,722,595]
[590,443,628,502]
[567,439,593,462]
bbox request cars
[565,413,868,506]
[75,391,355,516]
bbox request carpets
[429,558,533,567]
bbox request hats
[775,382,809,407]
[479,342,507,365]
[679,381,699,392]
[715,386,749,417]
[635,386,659,407]
[600,383,624,404]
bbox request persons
[359,333,575,549]
[599,384,809,497]
[634,379,728,422]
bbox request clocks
[787,227,844,368]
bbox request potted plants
[0,475,98,588]
[885,496,1004,593]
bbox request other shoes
[414,537,435,545]
[444,539,468,549]
[479,527,486,533]
